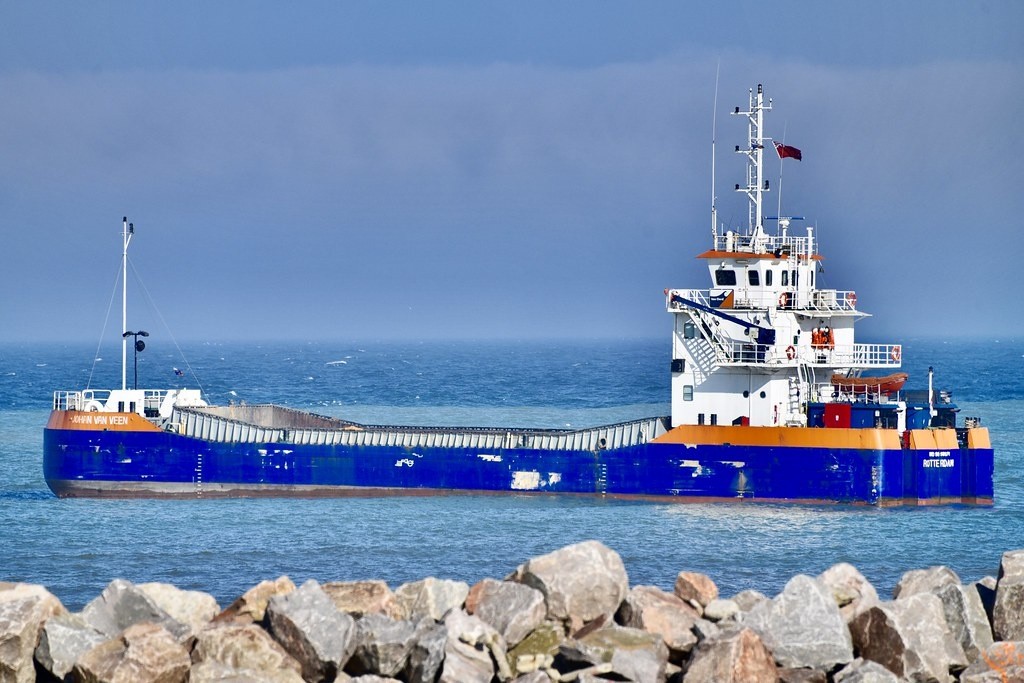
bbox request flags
[771,141,802,162]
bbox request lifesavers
[848,292,856,307]
[779,292,788,305]
[891,346,900,360]
[786,346,796,360]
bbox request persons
[169,425,175,432]
[599,439,607,450]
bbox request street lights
[123,331,149,389]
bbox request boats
[831,373,908,395]
[42,61,994,509]
